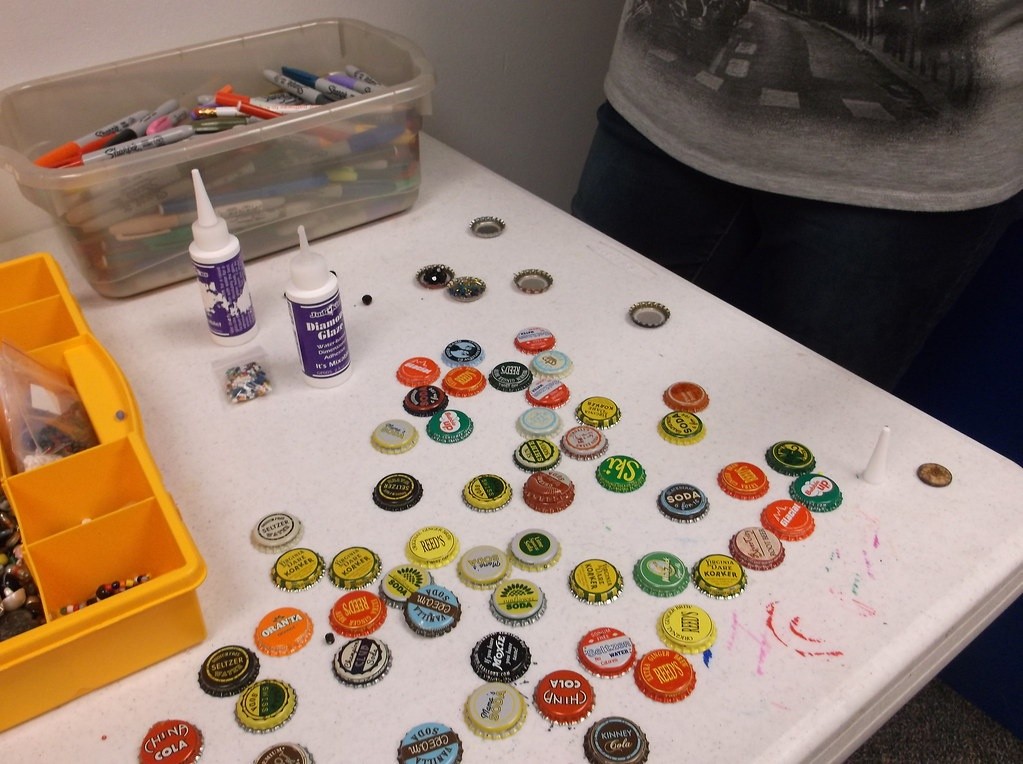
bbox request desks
[0,119,1023,764]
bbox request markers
[30,62,424,299]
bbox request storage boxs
[0,250,209,733]
[0,16,437,300]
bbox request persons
[567,0,1023,390]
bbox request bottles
[283,225,353,389]
[188,169,259,347]
